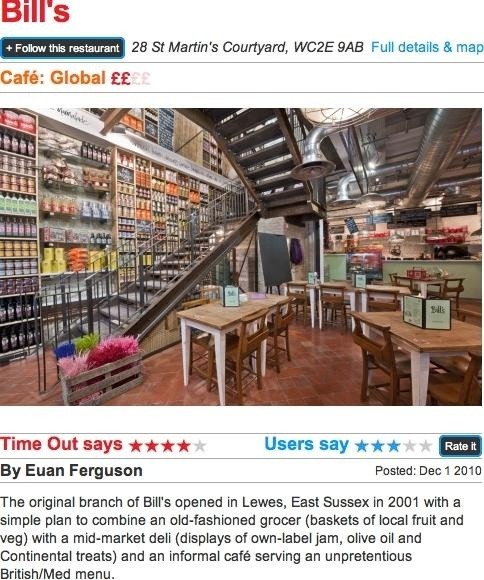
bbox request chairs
[430,308,482,379]
[366,288,399,312]
[286,282,311,329]
[389,273,399,286]
[350,310,436,406]
[207,310,270,405]
[249,297,292,373]
[428,350,482,406]
[430,276,465,317]
[318,284,347,335]
[181,300,241,393]
[393,275,420,311]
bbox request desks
[359,311,482,406]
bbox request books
[351,273,367,289]
[307,272,317,285]
[219,285,239,307]
[402,294,452,330]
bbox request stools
[335,299,351,320]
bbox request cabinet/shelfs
[0,108,137,370]
[136,154,222,273]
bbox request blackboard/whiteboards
[344,203,477,234]
[257,232,292,285]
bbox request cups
[239,294,248,302]
[41,247,66,273]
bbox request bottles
[89,232,111,244]
[78,140,113,164]
[41,194,77,214]
[0,127,35,156]
[117,148,227,267]
[0,296,42,353]
